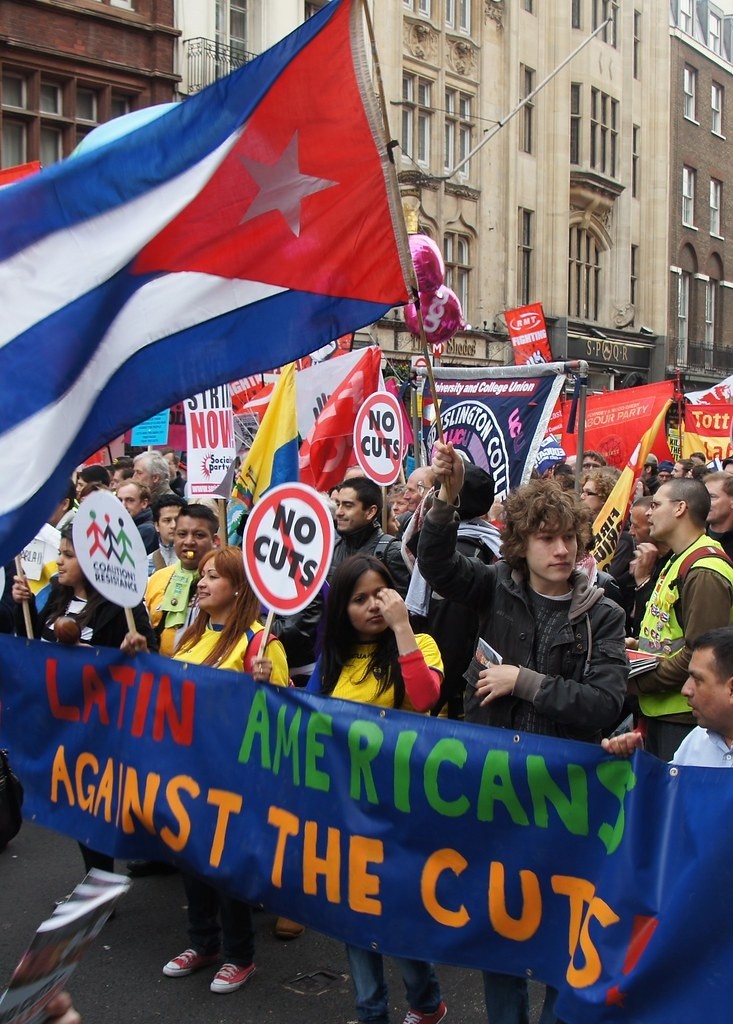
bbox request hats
[105,456,129,470]
[645,454,658,464]
[434,454,494,520]
[658,460,674,472]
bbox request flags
[298,348,382,492]
[1,0,415,566]
[232,363,299,509]
[589,400,671,573]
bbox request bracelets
[400,648,418,657]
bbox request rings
[261,668,262,673]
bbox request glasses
[582,464,599,469]
[417,481,432,497]
[579,489,599,496]
[650,499,688,511]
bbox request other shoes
[126,858,161,874]
[276,917,306,938]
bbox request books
[631,660,658,667]
[625,649,666,662]
[0,868,132,1024]
[476,638,503,669]
[629,662,660,678]
[630,657,661,663]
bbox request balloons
[404,236,467,346]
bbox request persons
[22,452,733,937]
[0,991,80,1024]
[408,442,628,1024]
[601,627,733,767]
[306,555,448,1024]
[12,529,149,921]
[127,503,216,876]
[121,548,289,992]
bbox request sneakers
[210,962,255,994]
[163,948,218,977]
[403,1000,447,1024]
